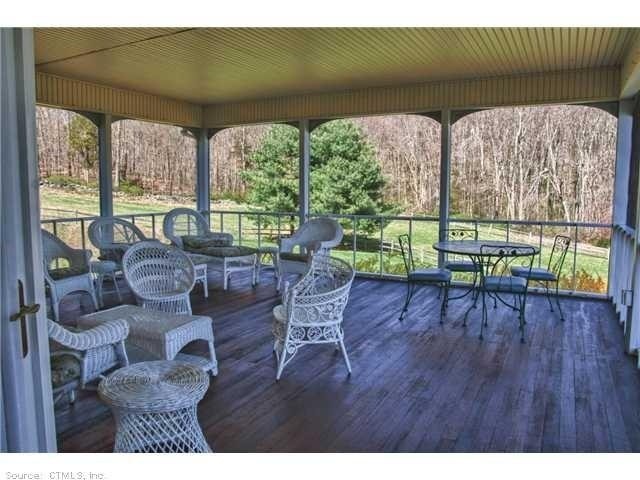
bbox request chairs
[39,207,356,403]
[398,228,573,343]
